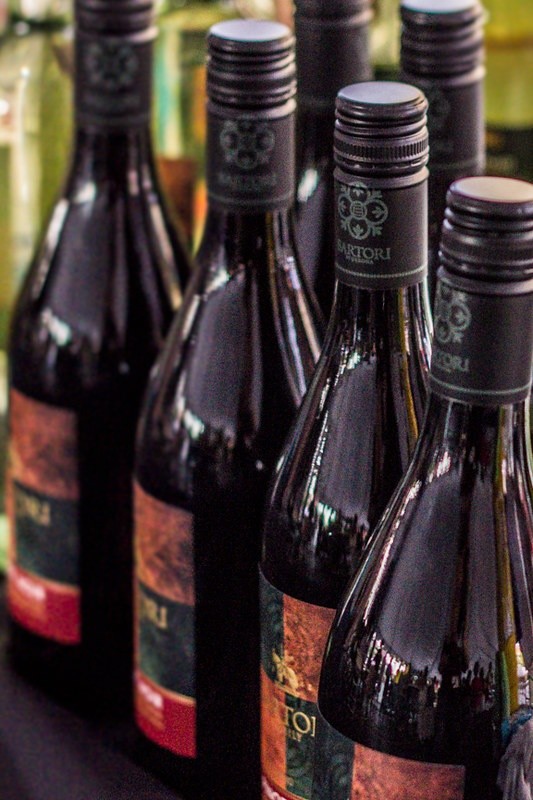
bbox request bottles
[1,0,532,454]
[127,18,325,795]
[257,81,435,800]
[317,175,533,800]
[4,0,194,708]
